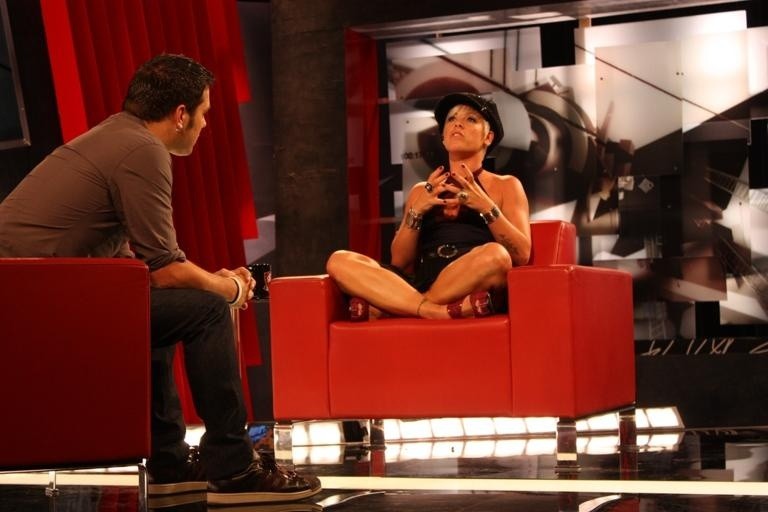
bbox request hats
[435,91,504,151]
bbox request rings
[459,191,467,201]
[425,183,433,192]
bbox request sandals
[447,289,494,319]
[349,298,370,321]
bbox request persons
[326,93,532,321]
[0,50,322,504]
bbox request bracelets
[480,206,500,225]
[406,208,423,230]
[228,276,242,306]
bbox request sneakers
[148,447,207,495]
[207,453,322,503]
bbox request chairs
[269,220,637,471]
[0,258,151,512]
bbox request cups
[248,264,272,301]
